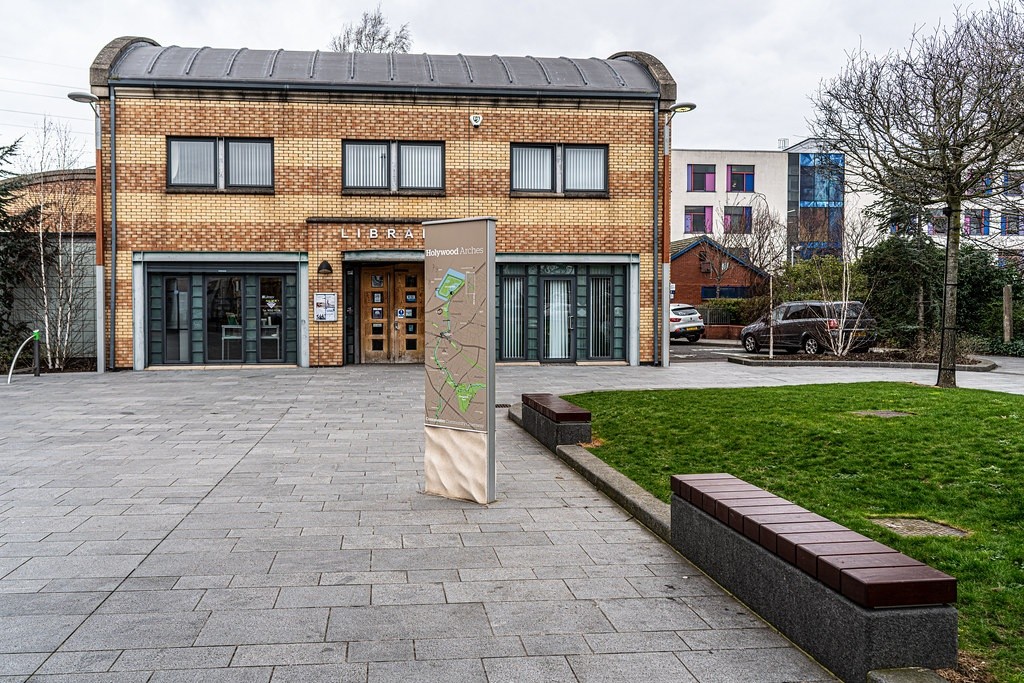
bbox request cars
[669,303,705,342]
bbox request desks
[221,324,280,360]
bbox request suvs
[739,300,878,356]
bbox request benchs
[669,473,960,683]
[521,393,592,454]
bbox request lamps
[317,261,333,274]
